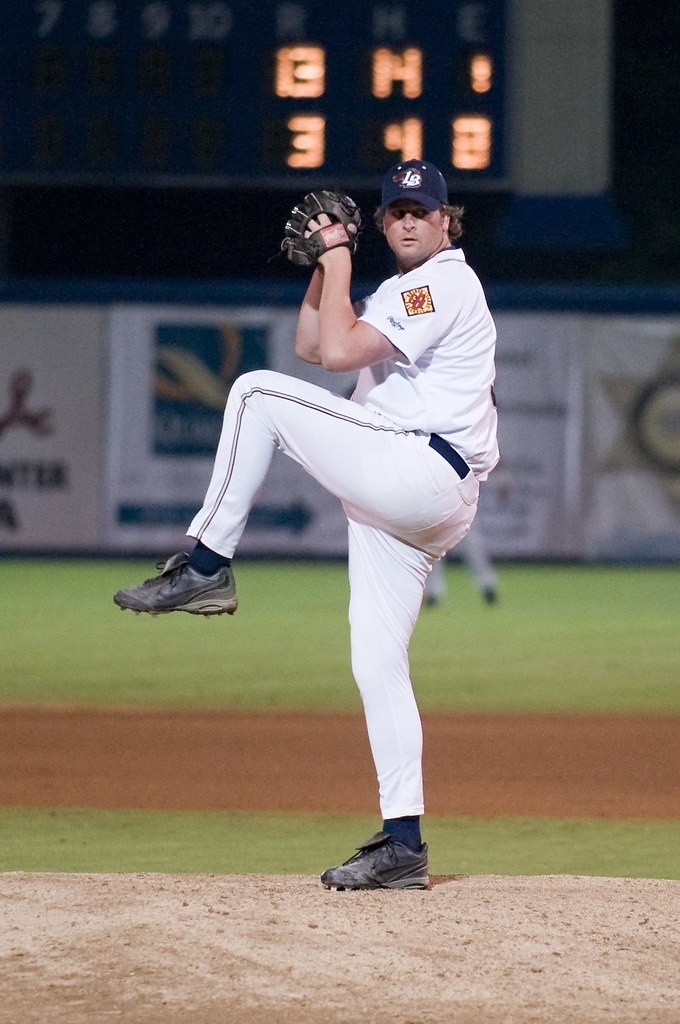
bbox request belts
[429,433,470,480]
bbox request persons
[114,158,499,890]
[423,526,500,606]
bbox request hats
[381,158,448,211]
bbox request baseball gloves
[280,188,365,269]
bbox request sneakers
[320,832,428,890]
[114,552,238,618]
